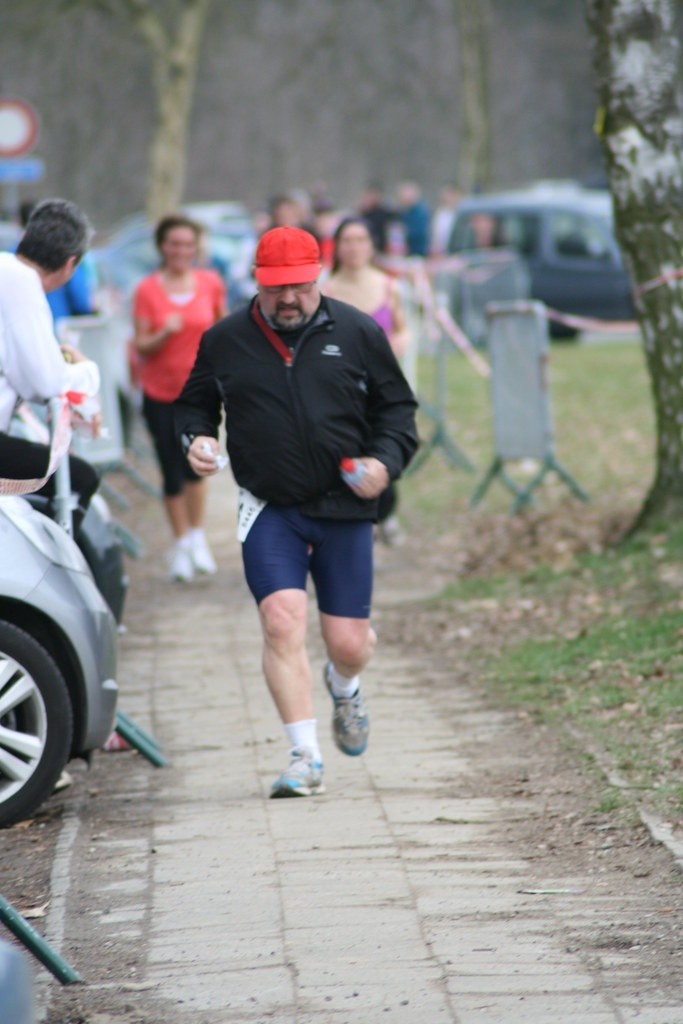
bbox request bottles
[340,458,366,488]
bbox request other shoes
[187,538,217,576]
[165,553,193,585]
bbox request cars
[0,398,129,829]
[444,196,643,349]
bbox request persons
[191,176,461,304]
[175,224,420,799]
[322,217,406,549]
[0,198,101,541]
[133,214,228,582]
[11,197,99,321]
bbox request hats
[253,225,321,287]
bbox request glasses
[262,281,316,294]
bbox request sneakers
[268,743,326,796]
[323,660,371,756]
